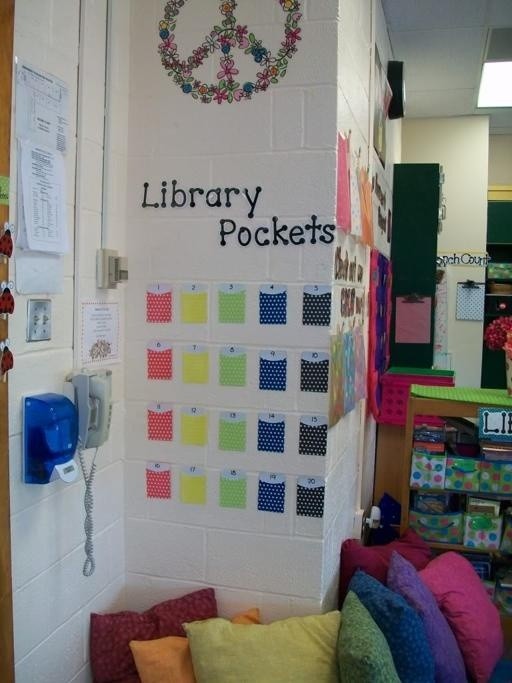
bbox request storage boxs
[406,447,512,553]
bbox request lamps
[475,23,512,111]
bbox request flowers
[481,313,511,358]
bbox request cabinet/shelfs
[387,161,441,368]
[371,364,457,519]
[399,383,510,656]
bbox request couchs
[86,541,512,683]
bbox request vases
[503,350,512,395]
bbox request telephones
[71,370,112,451]
